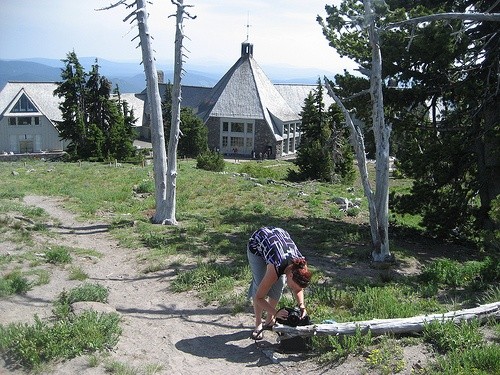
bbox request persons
[253,151,255,159]
[246,227,311,339]
[259,152,262,160]
[264,153,267,160]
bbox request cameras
[275,306,310,327]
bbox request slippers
[252,327,263,340]
[262,322,278,330]
